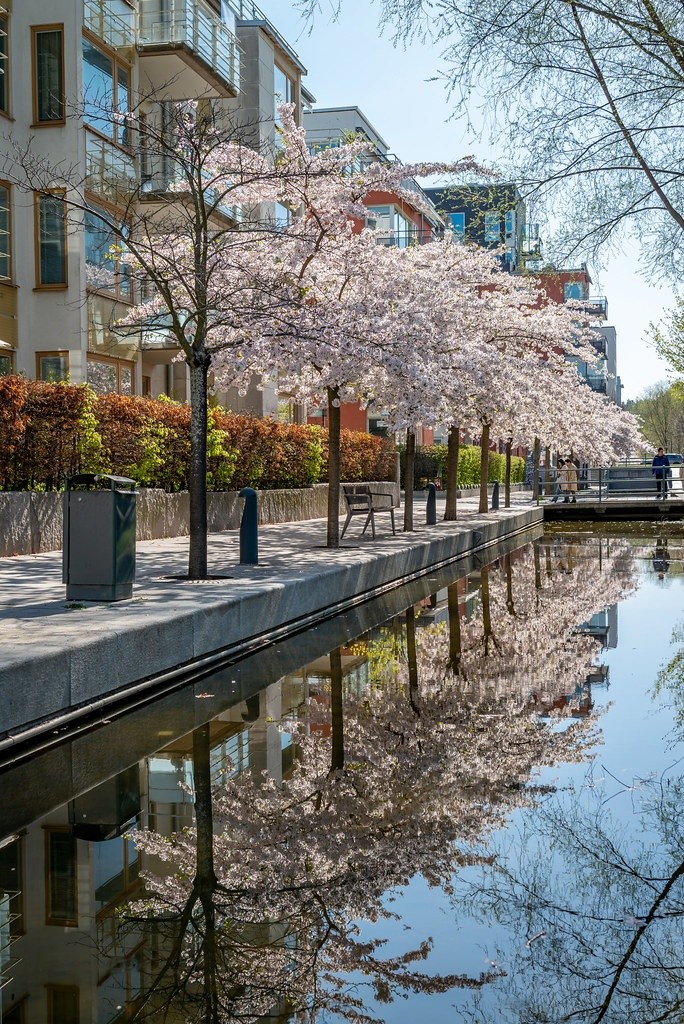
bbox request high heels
[551,499,557,503]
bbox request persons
[551,458,577,504]
[652,538,670,581]
[651,448,670,499]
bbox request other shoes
[657,494,661,497]
[562,500,568,503]
[665,495,667,498]
[570,500,576,503]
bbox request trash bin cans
[60,472,140,600]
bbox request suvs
[641,453,684,465]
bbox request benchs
[340,485,395,539]
